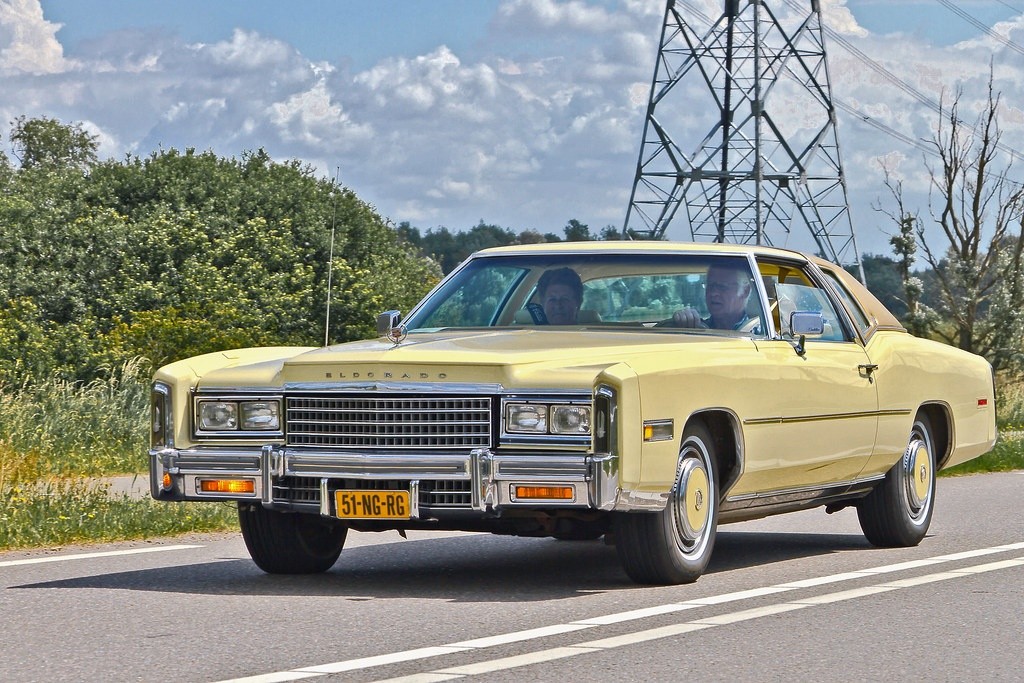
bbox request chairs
[769,299,795,333]
[513,311,601,323]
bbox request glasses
[543,295,578,303]
[702,283,737,289]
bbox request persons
[537,267,583,326]
[673,260,761,336]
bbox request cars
[147,241,998,587]
